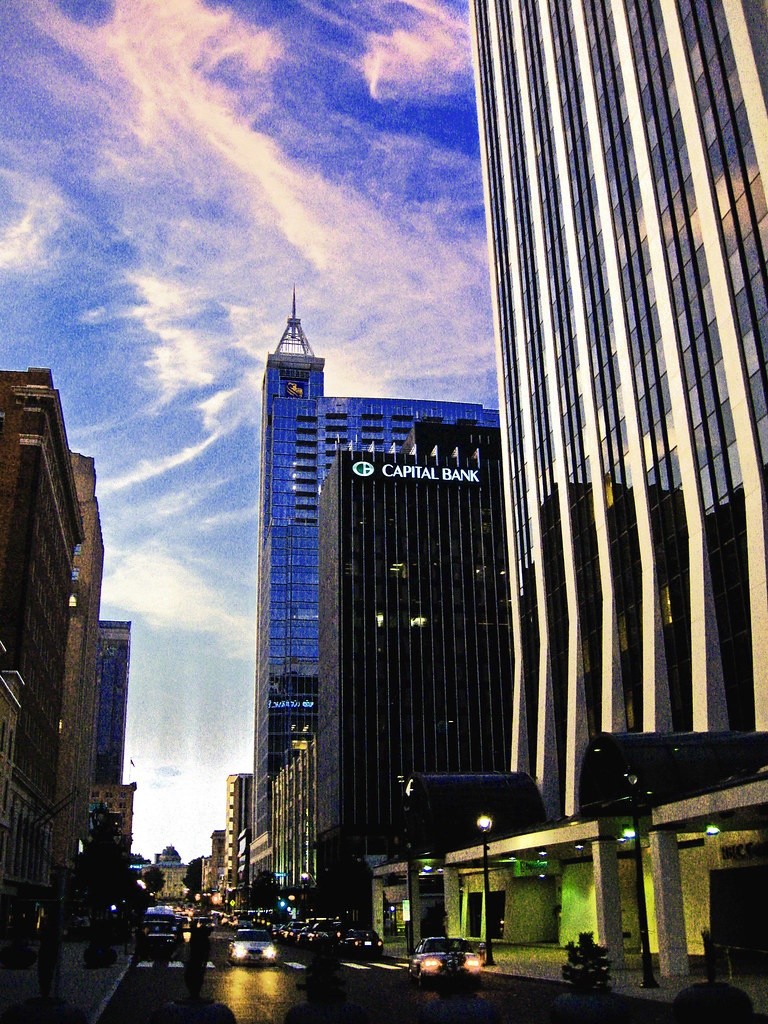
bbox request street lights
[477,815,497,967]
[301,873,310,919]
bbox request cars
[227,928,277,967]
[138,903,343,953]
[337,928,384,957]
[406,936,485,989]
